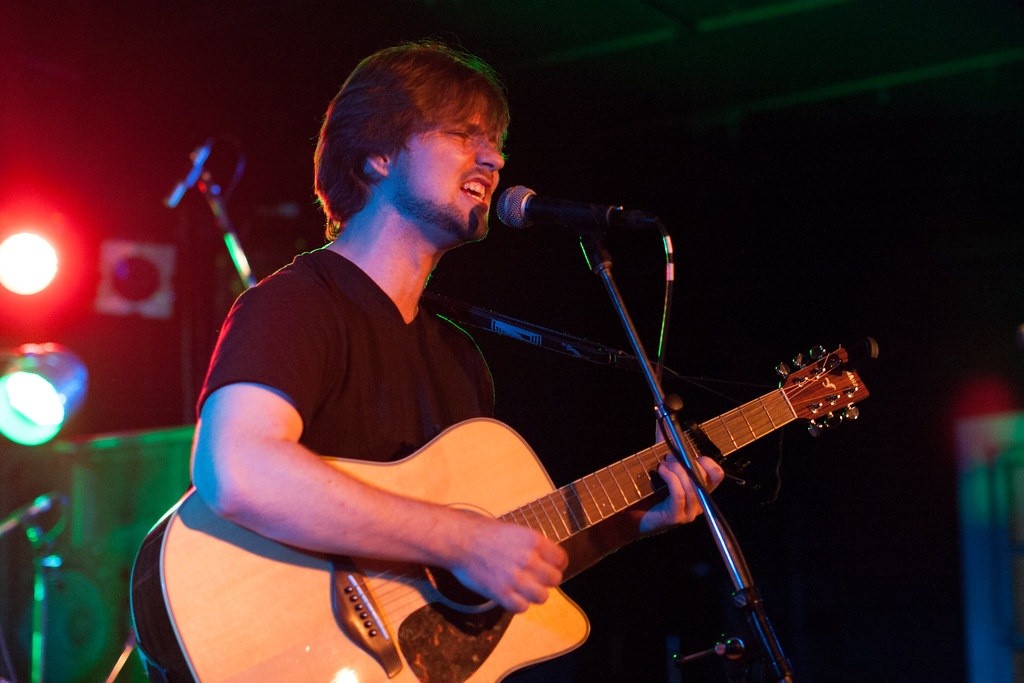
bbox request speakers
[40,423,198,683]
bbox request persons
[188,41,725,683]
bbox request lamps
[0,344,87,446]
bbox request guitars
[128,336,883,682]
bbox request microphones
[497,187,659,229]
[0,490,62,537]
[163,141,213,207]
